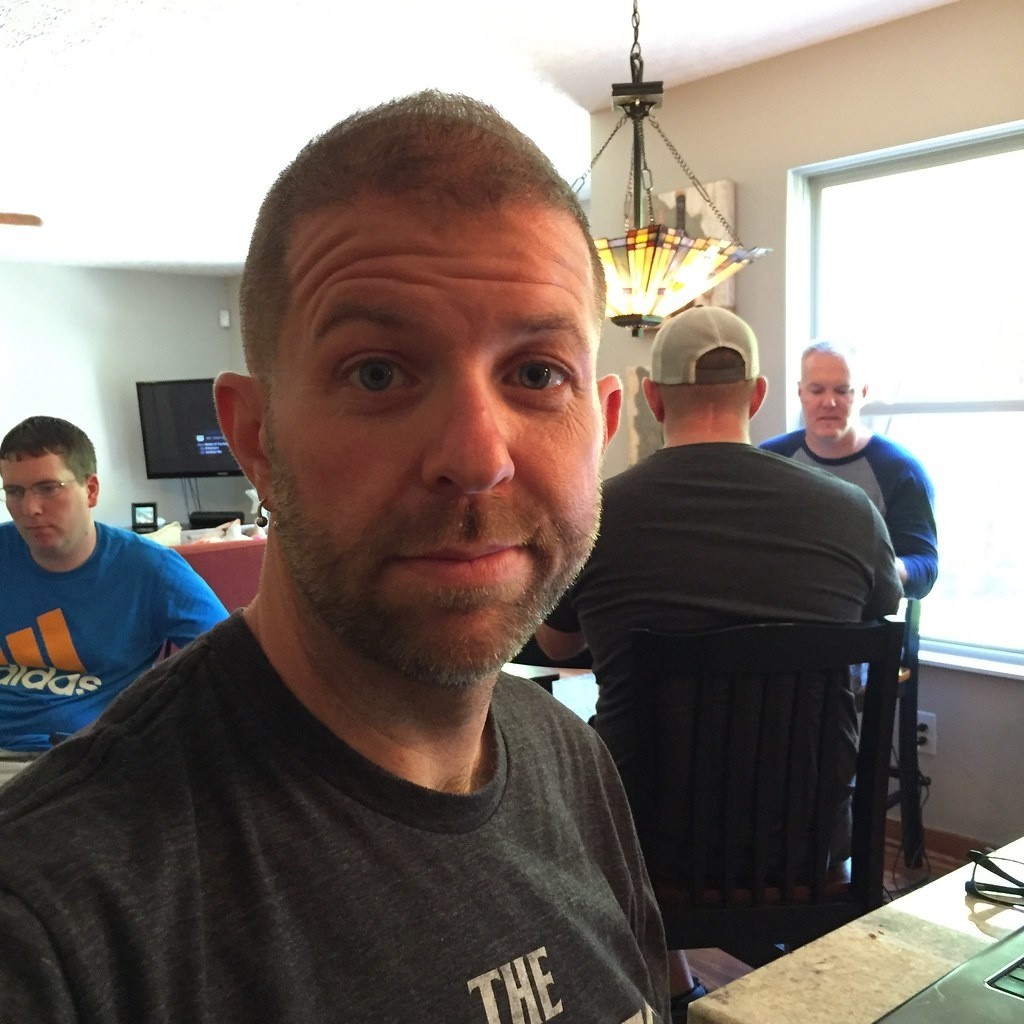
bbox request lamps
[566,0,777,338]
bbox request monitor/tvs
[135,378,246,479]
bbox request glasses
[965,850,1024,906]
[0,474,89,502]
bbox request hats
[649,304,760,385]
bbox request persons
[0,88,675,1024]
[0,416,232,788]
[756,342,939,695]
[534,306,904,1024]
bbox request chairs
[616,610,922,970]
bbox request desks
[683,833,1024,1024]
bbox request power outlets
[917,709,937,756]
